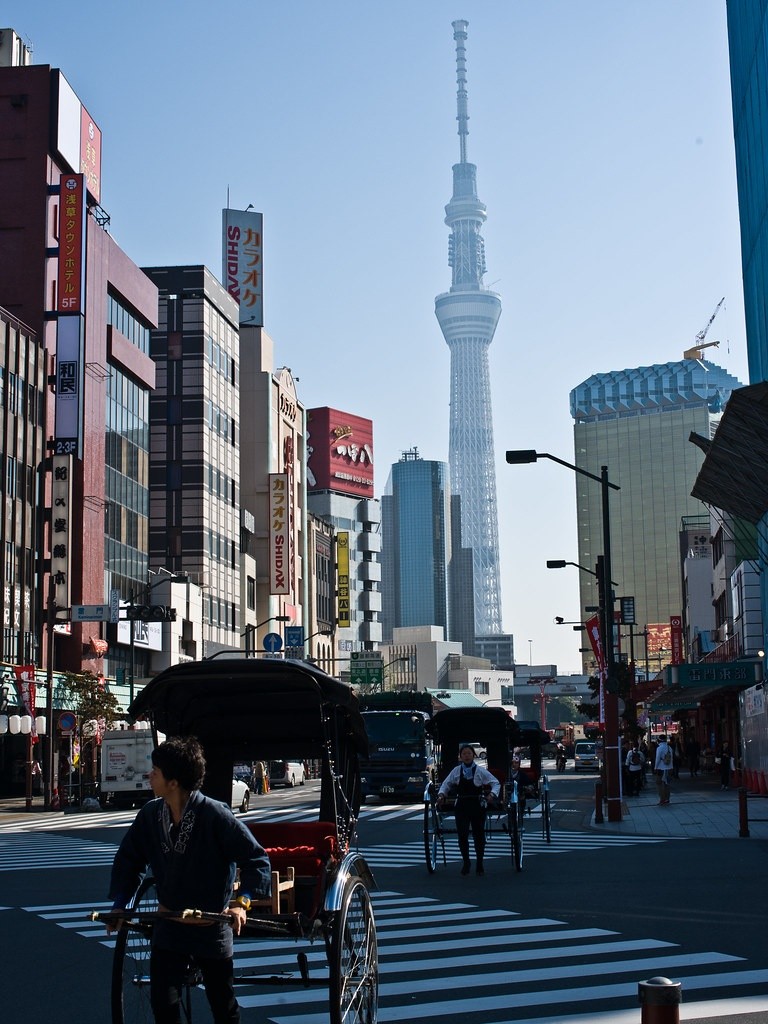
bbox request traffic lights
[0,686,9,713]
[126,605,173,622]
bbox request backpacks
[630,750,640,765]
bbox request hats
[659,735,666,741]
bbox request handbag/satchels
[675,743,679,757]
[664,751,671,764]
[715,757,721,764]
[730,757,736,771]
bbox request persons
[654,734,673,806]
[556,746,567,765]
[437,744,501,876]
[254,761,264,795]
[667,736,700,779]
[622,739,648,797]
[105,740,272,1024]
[505,756,528,832]
[715,740,734,791]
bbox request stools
[233,866,296,915]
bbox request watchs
[236,896,251,910]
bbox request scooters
[556,753,567,772]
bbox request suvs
[541,740,568,759]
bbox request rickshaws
[507,720,556,843]
[90,650,382,1024]
[416,705,524,875]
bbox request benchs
[246,821,349,885]
[522,767,539,789]
[439,769,508,805]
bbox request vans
[573,742,601,771]
[268,760,307,786]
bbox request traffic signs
[351,651,384,684]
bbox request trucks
[98,730,171,811]
[351,709,440,806]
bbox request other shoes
[724,787,728,792]
[659,798,668,807]
[517,827,526,832]
[475,860,485,875]
[720,786,724,791]
[461,861,471,876]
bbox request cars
[231,772,251,814]
[468,743,487,759]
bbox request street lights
[526,675,557,736]
[546,557,622,799]
[122,575,191,732]
[503,449,623,826]
[240,616,291,659]
[528,638,532,670]
